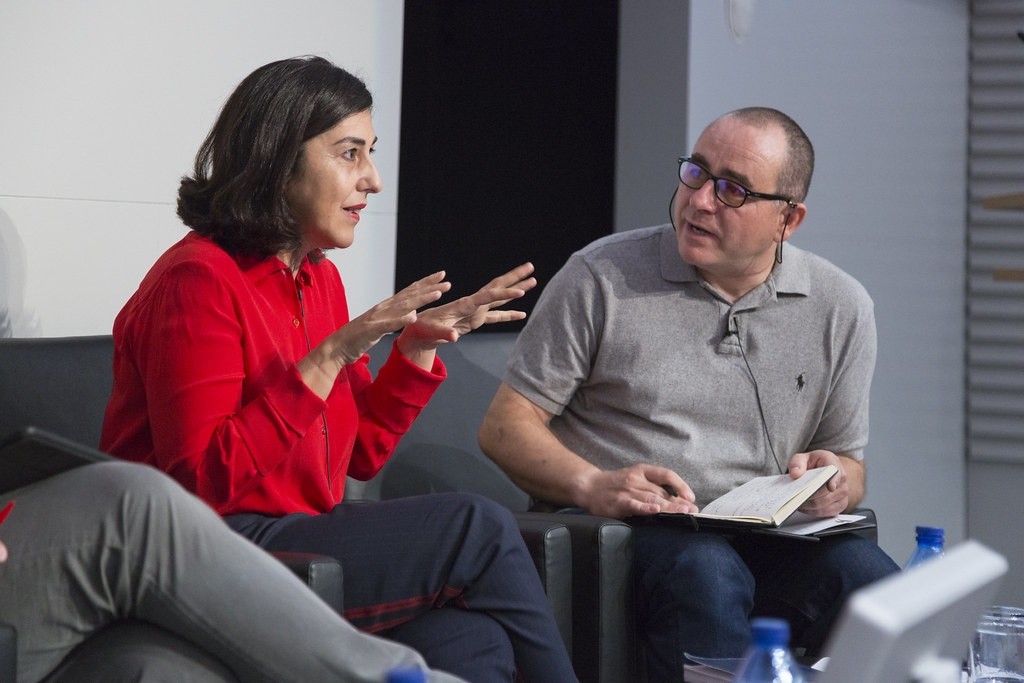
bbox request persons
[100,56,577,683]
[476,106,901,682]
[0,461,467,683]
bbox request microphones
[733,316,739,327]
[299,290,303,302]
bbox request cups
[970,606,1024,683]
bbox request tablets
[0,424,117,495]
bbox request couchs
[0,331,878,683]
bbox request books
[624,464,876,543]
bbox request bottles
[907,525,947,567]
[735,617,802,682]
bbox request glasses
[678,156,794,208]
